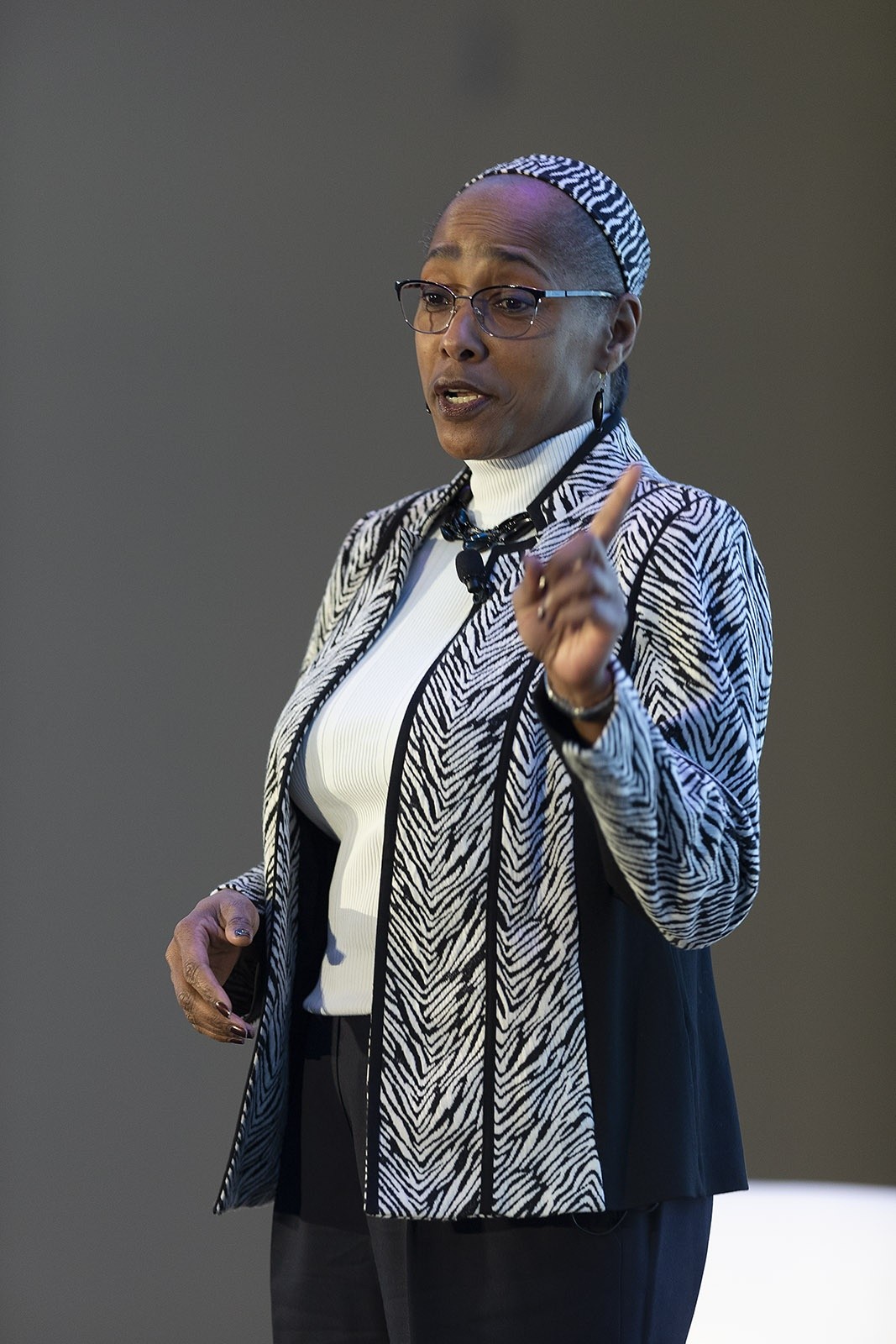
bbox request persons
[164,151,772,1343]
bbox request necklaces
[438,502,534,556]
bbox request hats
[456,152,653,300]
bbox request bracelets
[542,672,611,719]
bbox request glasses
[394,280,620,339]
